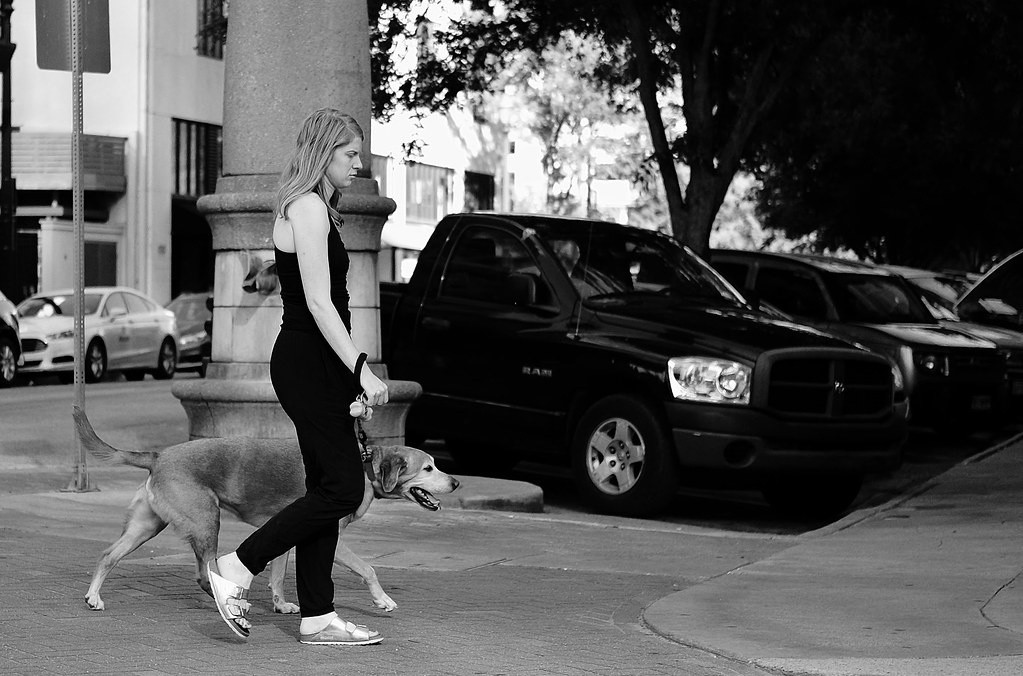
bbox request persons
[553,240,599,298]
[207,110,390,647]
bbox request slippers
[206,558,251,639]
[300,616,385,645]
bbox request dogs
[72,405,459,613]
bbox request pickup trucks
[375,212,912,525]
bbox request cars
[163,294,211,376]
[13,287,180,383]
[0,292,21,385]
[631,246,1023,472]
